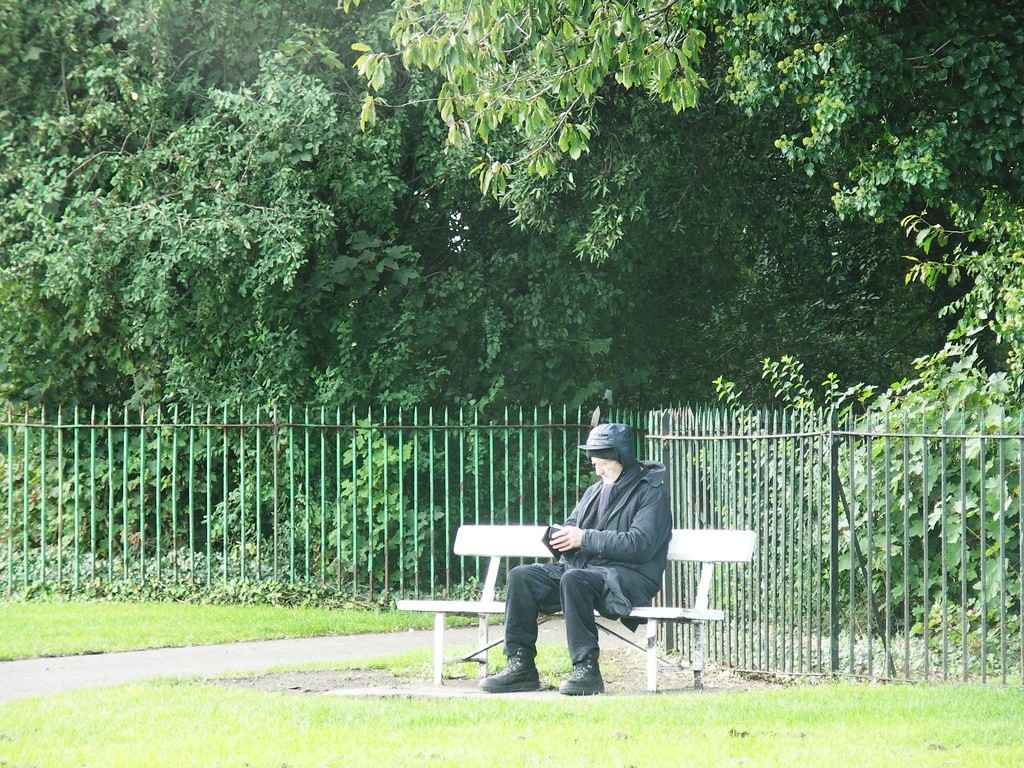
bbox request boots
[479,647,540,693]
[560,648,604,695]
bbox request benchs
[397,524,759,692]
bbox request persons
[479,422,672,695]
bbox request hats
[577,423,635,465]
[587,447,622,464]
